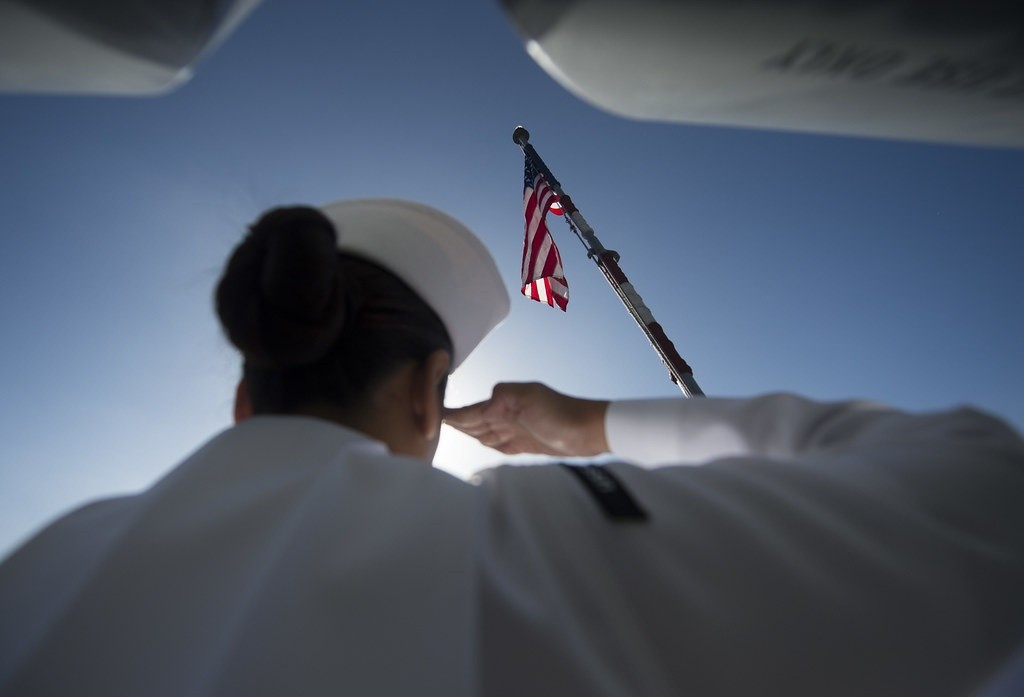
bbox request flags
[520,143,570,312]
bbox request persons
[0,200,1024,697]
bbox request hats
[317,196,510,375]
